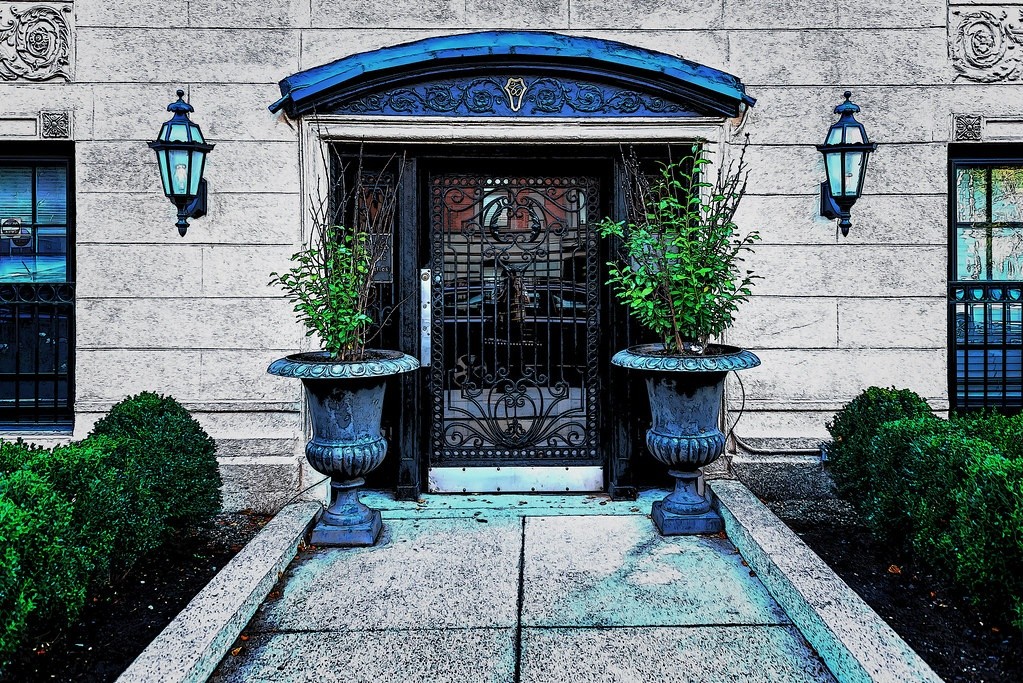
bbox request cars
[442,282,590,387]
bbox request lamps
[815,90,878,238]
[146,89,217,237]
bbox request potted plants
[592,130,760,535]
[266,98,426,549]
